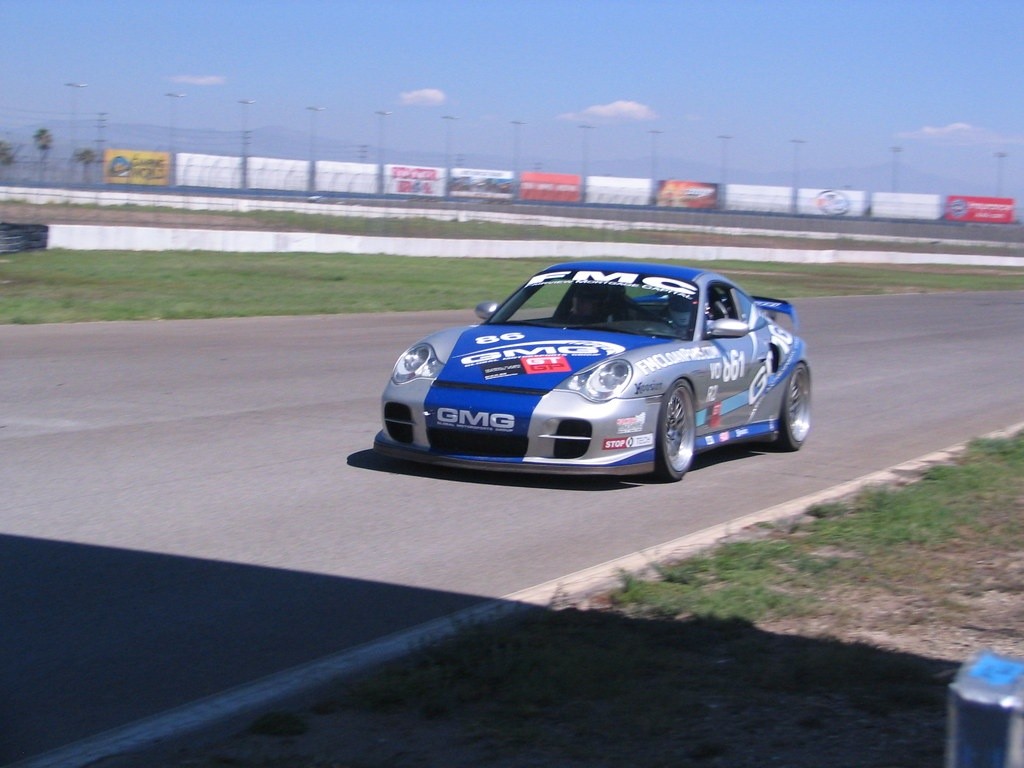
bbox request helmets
[669,295,691,326]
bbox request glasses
[584,294,606,300]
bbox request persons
[668,290,697,336]
[568,289,603,322]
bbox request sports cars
[371,255,814,483]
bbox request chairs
[604,285,629,322]
